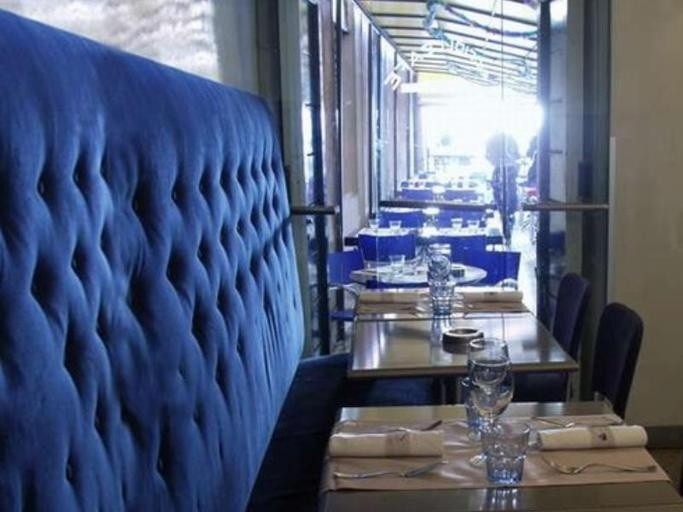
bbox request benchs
[0,3,447,509]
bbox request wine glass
[461,336,514,469]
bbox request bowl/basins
[442,328,485,353]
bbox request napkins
[330,430,444,458]
[537,426,648,450]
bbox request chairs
[328,169,522,321]
[590,303,644,422]
[504,272,592,402]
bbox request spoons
[334,459,448,478]
[531,414,623,428]
[541,452,659,474]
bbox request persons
[485,119,518,241]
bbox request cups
[428,243,455,318]
[480,484,523,512]
[388,254,405,269]
[368,217,479,235]
[476,422,531,485]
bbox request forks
[340,419,442,433]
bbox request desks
[349,285,580,403]
[321,407,681,512]
